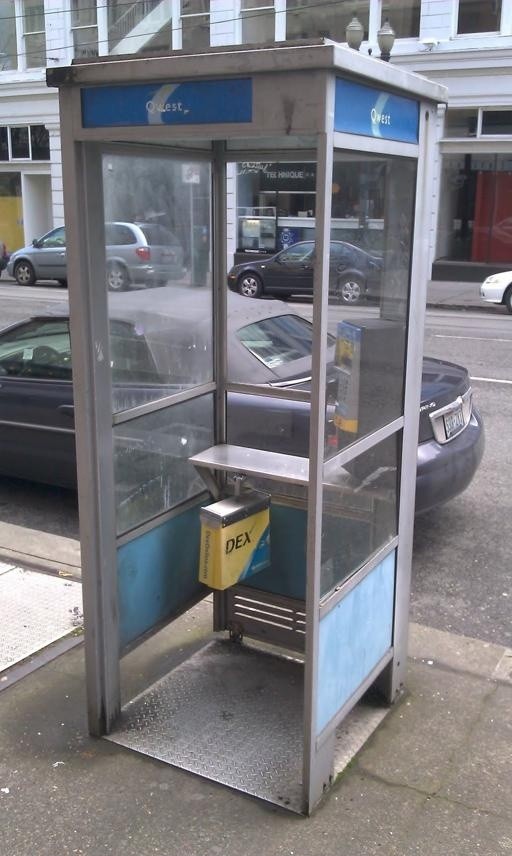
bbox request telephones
[324,318,404,485]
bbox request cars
[0,221,187,291]
[0,288,485,522]
[478,270,511,311]
[227,240,398,306]
